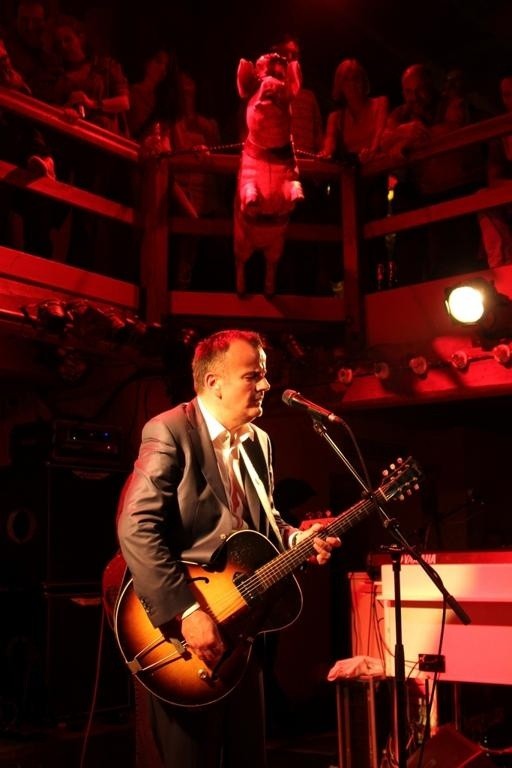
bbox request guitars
[117,457,421,706]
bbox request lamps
[31,296,306,364]
[441,278,496,328]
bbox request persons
[1,2,510,296]
[112,325,344,768]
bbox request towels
[325,655,386,683]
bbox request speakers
[405,722,512,768]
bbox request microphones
[281,389,340,423]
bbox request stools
[335,669,384,768]
